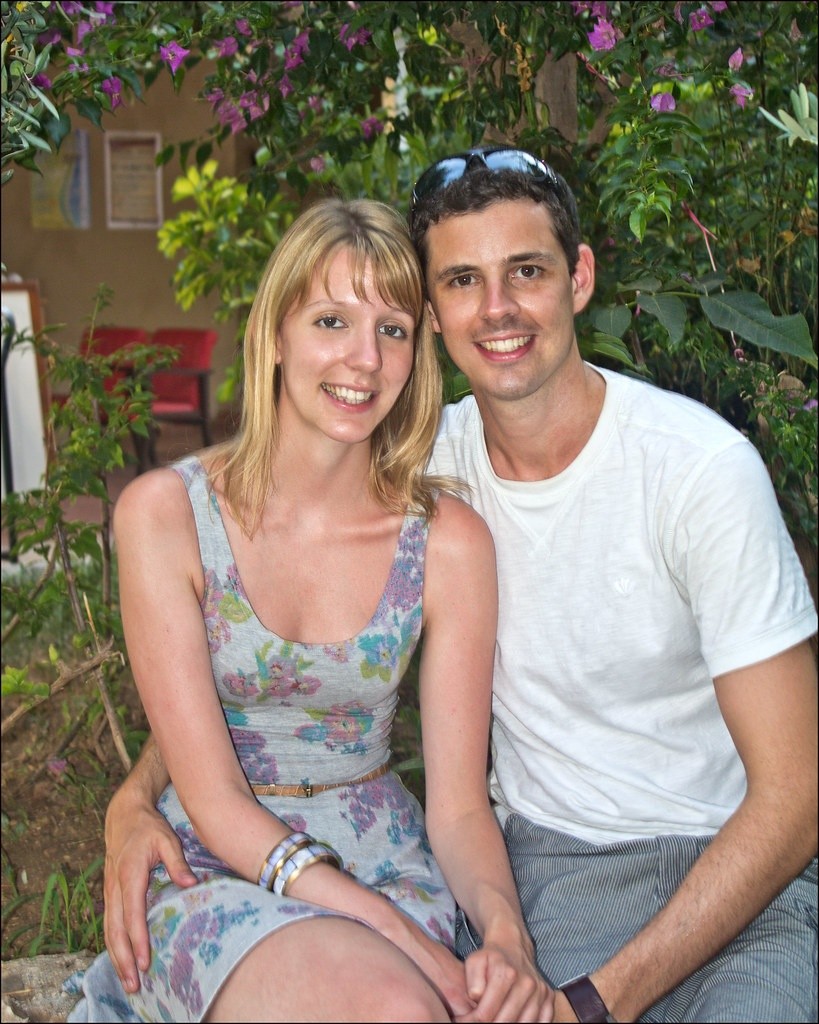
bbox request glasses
[409,148,575,232]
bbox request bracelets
[258,831,341,898]
[560,973,616,1024]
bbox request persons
[71,197,557,1023]
[104,146,819,1024]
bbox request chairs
[51,326,160,471]
[103,328,215,475]
